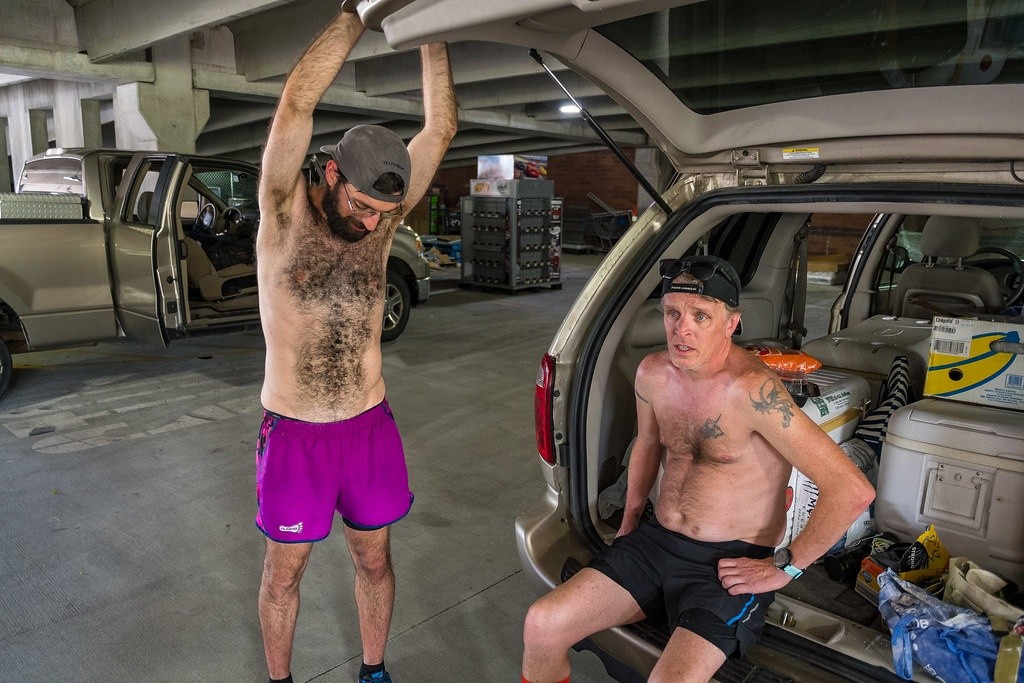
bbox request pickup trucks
[0,147,431,402]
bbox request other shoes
[357,670,393,683]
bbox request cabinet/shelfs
[459,196,563,296]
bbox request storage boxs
[791,438,878,564]
[922,315,1024,411]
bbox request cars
[341,1,1024,683]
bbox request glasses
[659,257,731,282]
[341,183,404,219]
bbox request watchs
[772,546,805,579]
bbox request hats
[661,254,743,337]
[320,124,412,202]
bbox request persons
[254,0,456,683]
[523,256,875,683]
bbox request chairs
[890,215,1001,318]
[137,191,258,300]
